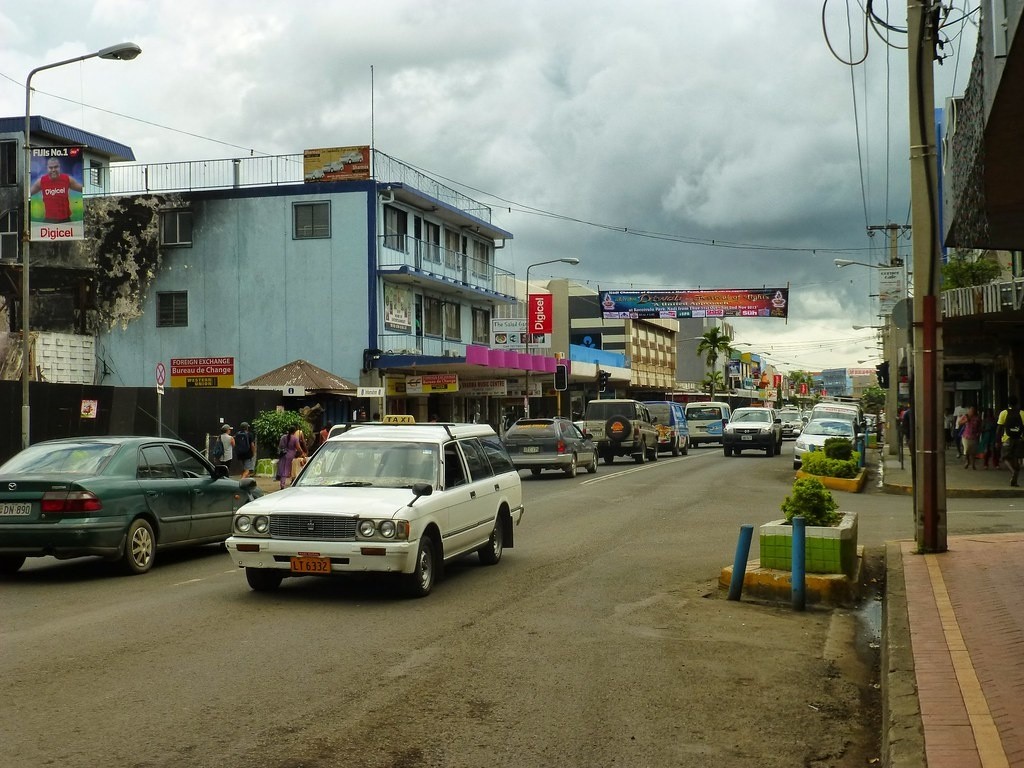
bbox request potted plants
[794,436,866,493]
[759,475,859,574]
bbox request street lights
[525,257,580,418]
[21,41,144,453]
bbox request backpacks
[235,431,253,460]
[1005,408,1024,439]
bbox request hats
[221,424,233,430]
[240,422,251,429]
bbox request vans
[685,402,731,449]
[641,401,690,457]
[776,404,812,438]
[801,396,866,432]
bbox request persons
[277,420,307,489]
[373,413,380,422]
[30,156,83,224]
[320,424,329,444]
[213,423,235,468]
[235,422,256,478]
[898,402,911,456]
[944,395,1024,486]
[430,414,438,423]
[359,410,366,419]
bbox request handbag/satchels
[299,430,305,450]
[957,413,968,437]
[211,434,224,458]
[275,436,287,457]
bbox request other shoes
[1010,470,1019,486]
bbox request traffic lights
[554,364,568,392]
[599,370,611,393]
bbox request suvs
[722,406,782,457]
[583,398,659,465]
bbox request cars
[502,415,599,478]
[305,151,368,181]
[793,417,865,471]
[863,413,878,432]
[224,413,525,598]
[0,435,264,577]
